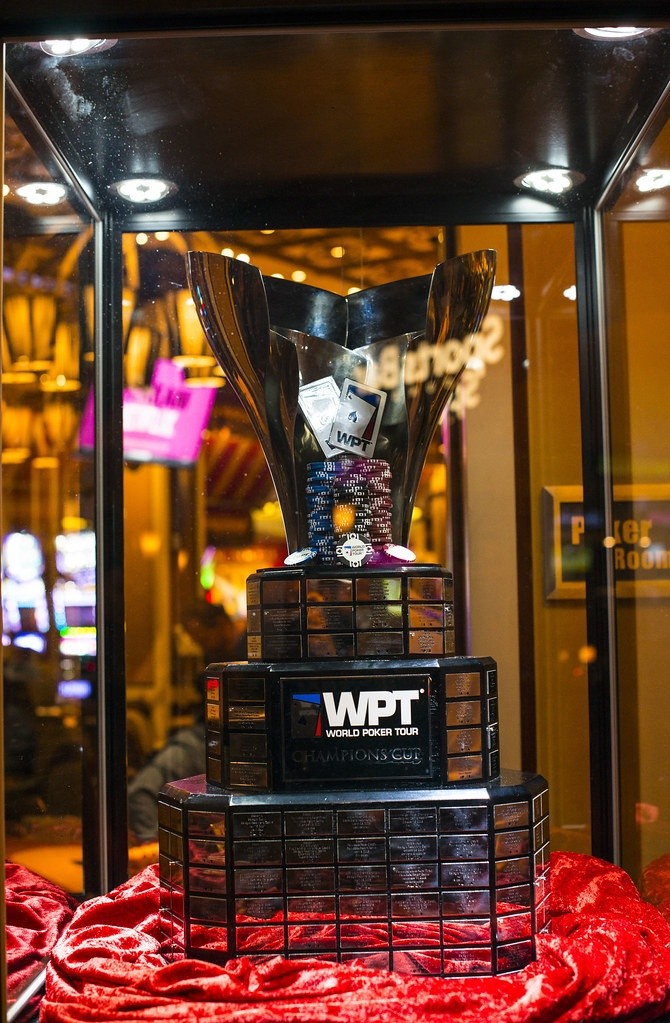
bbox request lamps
[0,230,228,470]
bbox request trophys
[155,248,553,975]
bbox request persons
[127,599,248,845]
